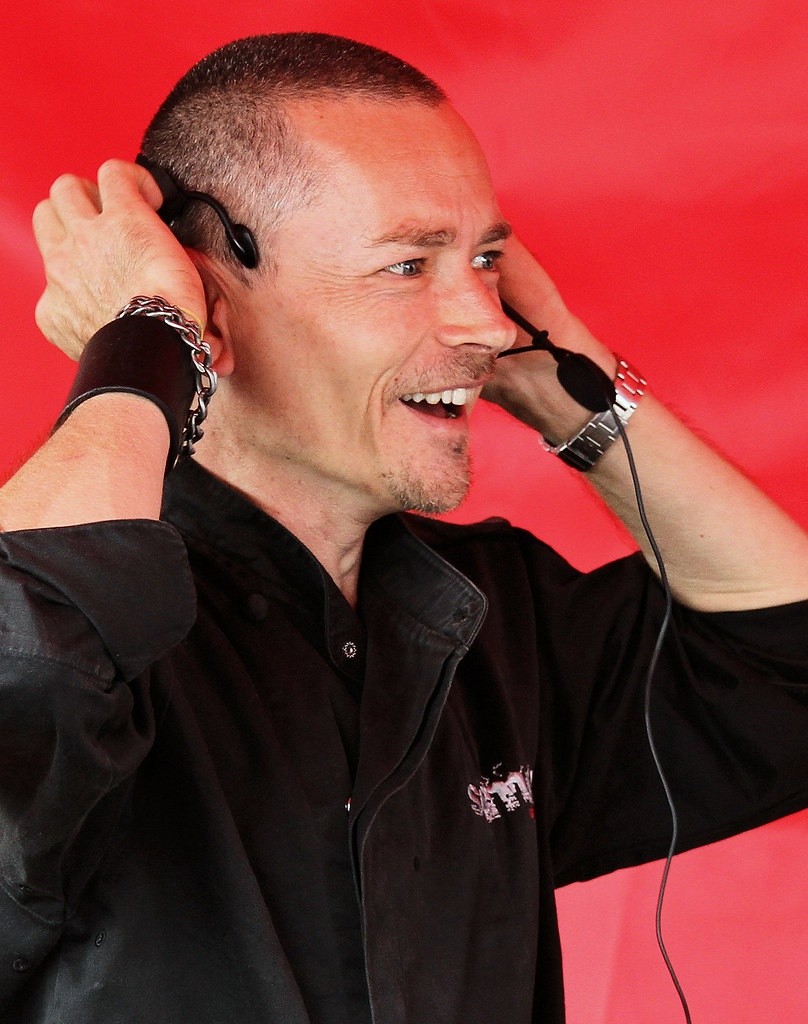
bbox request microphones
[499,299,616,413]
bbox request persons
[0,34,808,1024]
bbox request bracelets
[539,353,648,472]
[45,313,199,477]
[111,294,218,474]
